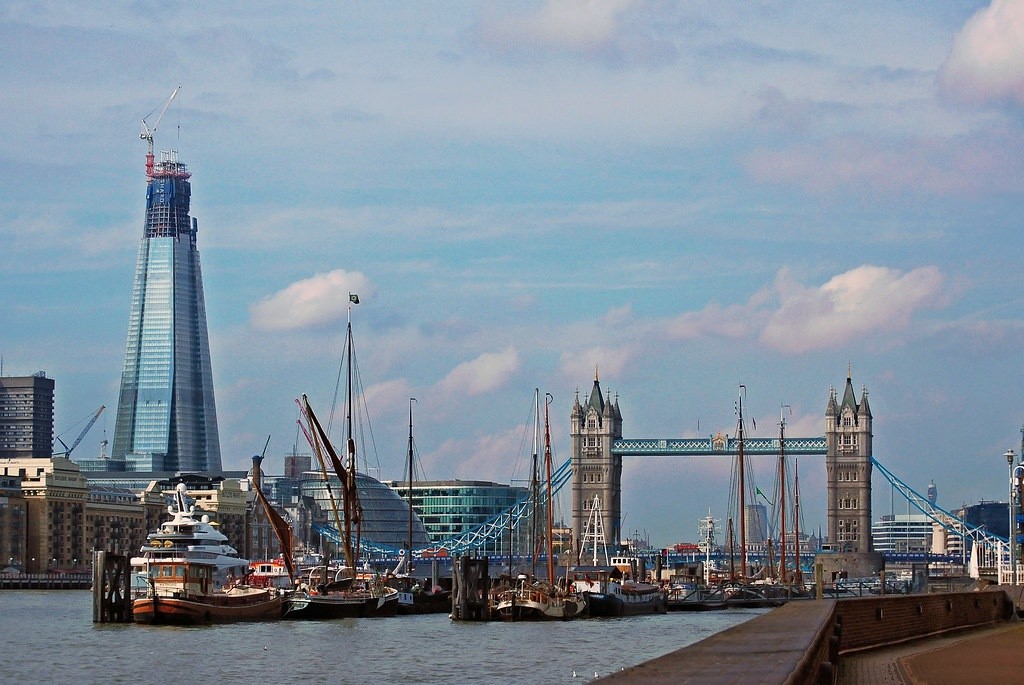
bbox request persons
[834,571,846,589]
[674,577,681,583]
[267,577,273,587]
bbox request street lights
[1003,449,1019,585]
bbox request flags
[753,418,757,430]
[350,295,361,305]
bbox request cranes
[139,85,190,179]
[52,405,109,458]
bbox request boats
[102,292,819,622]
